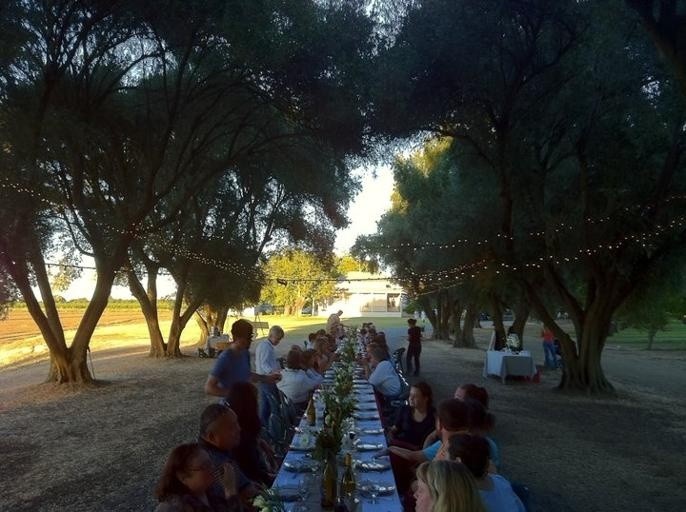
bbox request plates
[356,415,381,420]
[284,457,314,473]
[356,458,391,472]
[276,484,300,502]
[356,442,384,451]
[361,426,384,434]
[360,479,396,496]
[289,443,315,452]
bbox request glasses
[223,401,230,414]
[189,462,214,474]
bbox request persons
[254,325,281,427]
[412,461,482,511]
[226,382,278,488]
[441,435,525,511]
[155,443,214,512]
[198,404,263,512]
[360,322,387,350]
[291,329,337,378]
[361,349,401,407]
[387,381,499,472]
[539,324,557,369]
[273,351,318,412]
[205,319,266,412]
[405,318,423,376]
[326,311,348,340]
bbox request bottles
[307,391,317,431]
[322,456,338,510]
[341,455,356,510]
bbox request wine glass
[366,478,380,504]
[298,480,312,502]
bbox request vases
[321,454,337,508]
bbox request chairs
[277,389,302,427]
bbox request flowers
[301,414,343,459]
[251,480,309,512]
[339,326,357,362]
[506,332,520,349]
[330,361,354,400]
[313,383,358,425]
